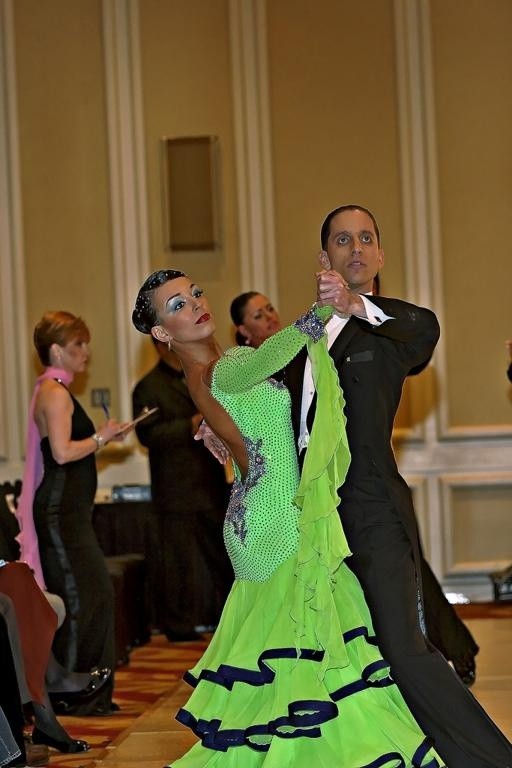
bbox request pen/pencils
[101,403,110,420]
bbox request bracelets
[91,434,106,451]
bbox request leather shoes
[31,726,89,754]
[48,668,111,712]
[453,655,476,688]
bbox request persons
[22,307,123,717]
[127,326,232,642]
[0,491,94,754]
[418,557,481,688]
[193,202,511,768]
[129,266,444,768]
[229,289,315,473]
